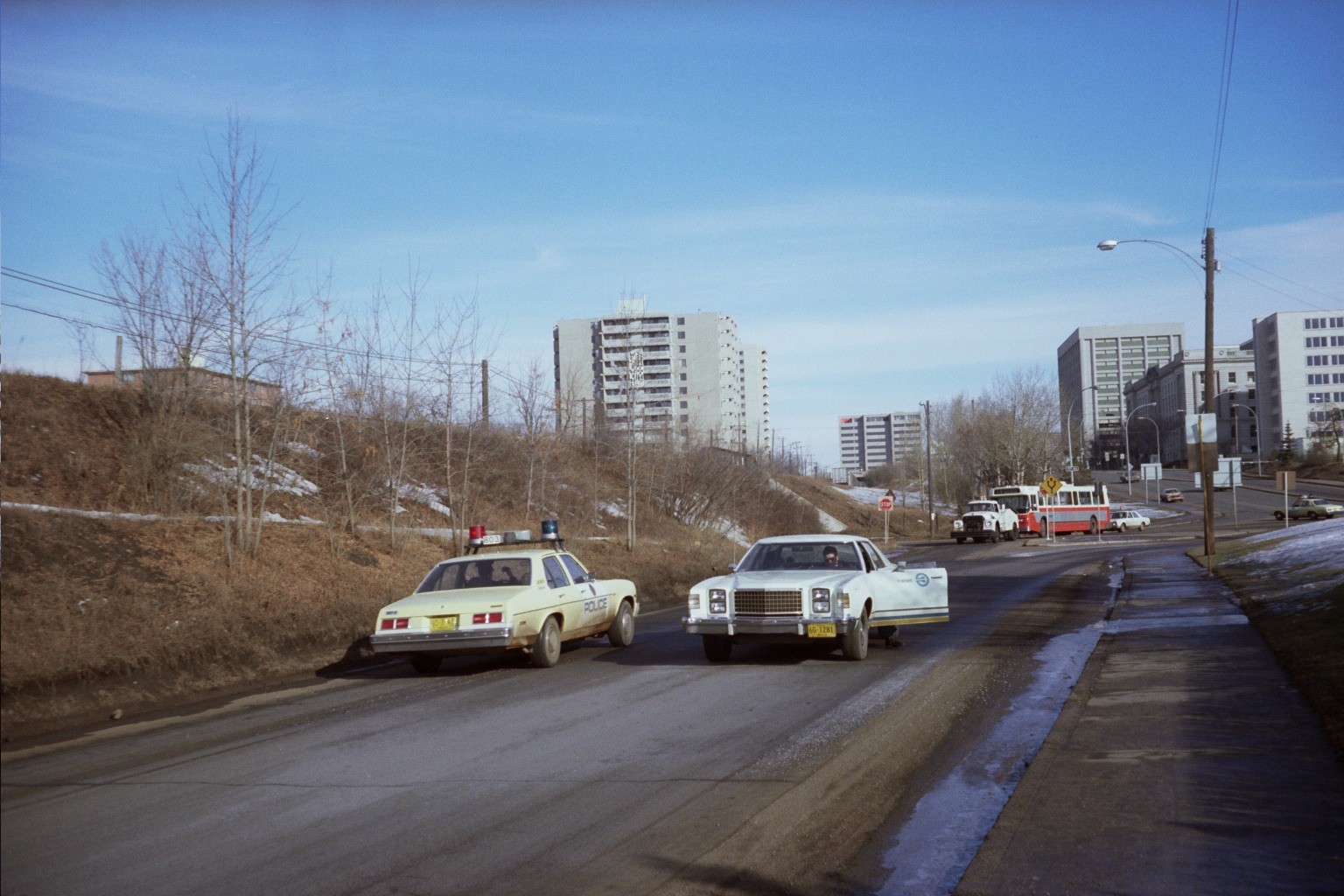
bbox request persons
[479,560,518,586]
[823,546,838,567]
